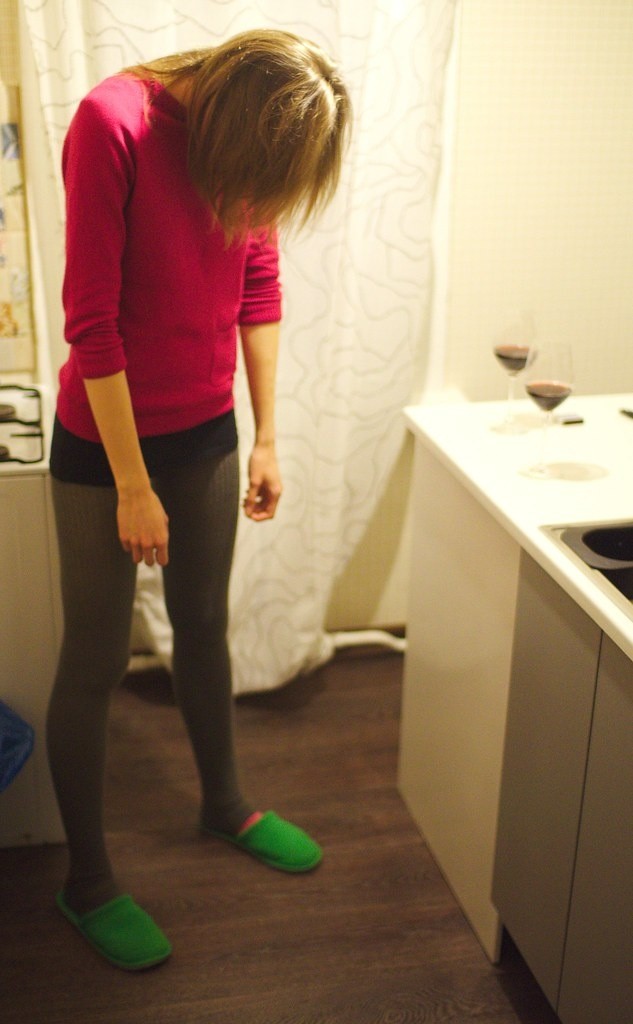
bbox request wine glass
[492,338,533,432]
[523,378,574,477]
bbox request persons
[46,30,353,971]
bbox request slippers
[57,887,171,971]
[198,808,321,874]
